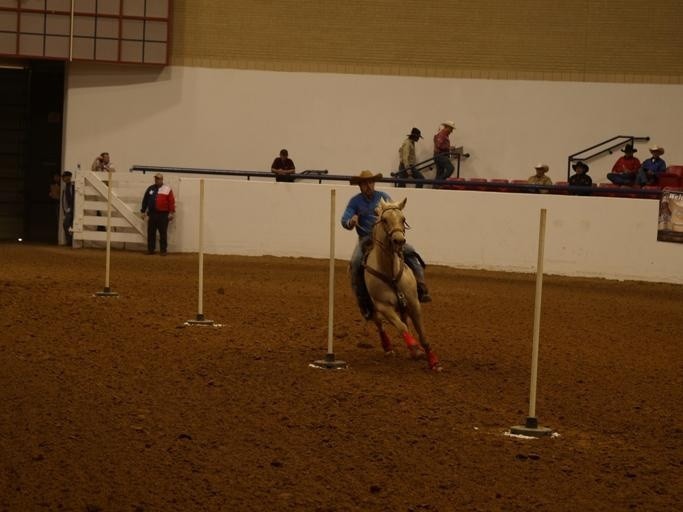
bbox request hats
[649,144,665,156]
[406,128,424,139]
[440,120,456,129]
[61,171,72,177]
[621,143,638,154]
[350,169,383,186]
[153,173,164,179]
[534,162,550,173]
[280,149,288,156]
[572,160,589,173]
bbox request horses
[364,194,444,373]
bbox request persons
[432,120,456,187]
[138,173,176,257]
[90,151,116,232]
[396,126,425,188]
[269,148,295,183]
[339,170,432,324]
[526,162,553,186]
[61,170,76,248]
[567,160,592,186]
[635,143,667,187]
[606,142,640,185]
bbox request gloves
[140,211,148,221]
[168,210,175,224]
[349,215,360,229]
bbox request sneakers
[144,249,156,256]
[160,249,167,257]
[62,242,72,247]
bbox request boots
[352,277,377,321]
[416,280,433,304]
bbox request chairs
[443,163,682,201]
